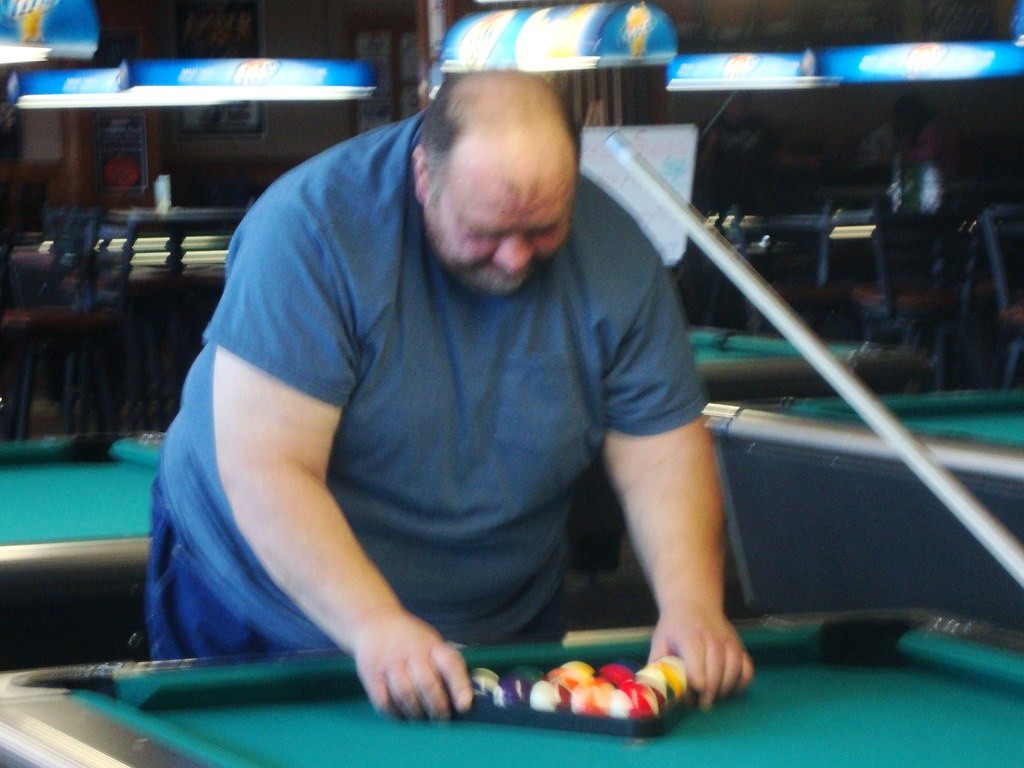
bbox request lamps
[0,0,1024,109]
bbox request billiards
[459,654,689,725]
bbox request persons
[695,89,967,184]
[144,69,755,722]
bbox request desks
[0,327,1024,768]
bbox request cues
[599,129,1024,593]
